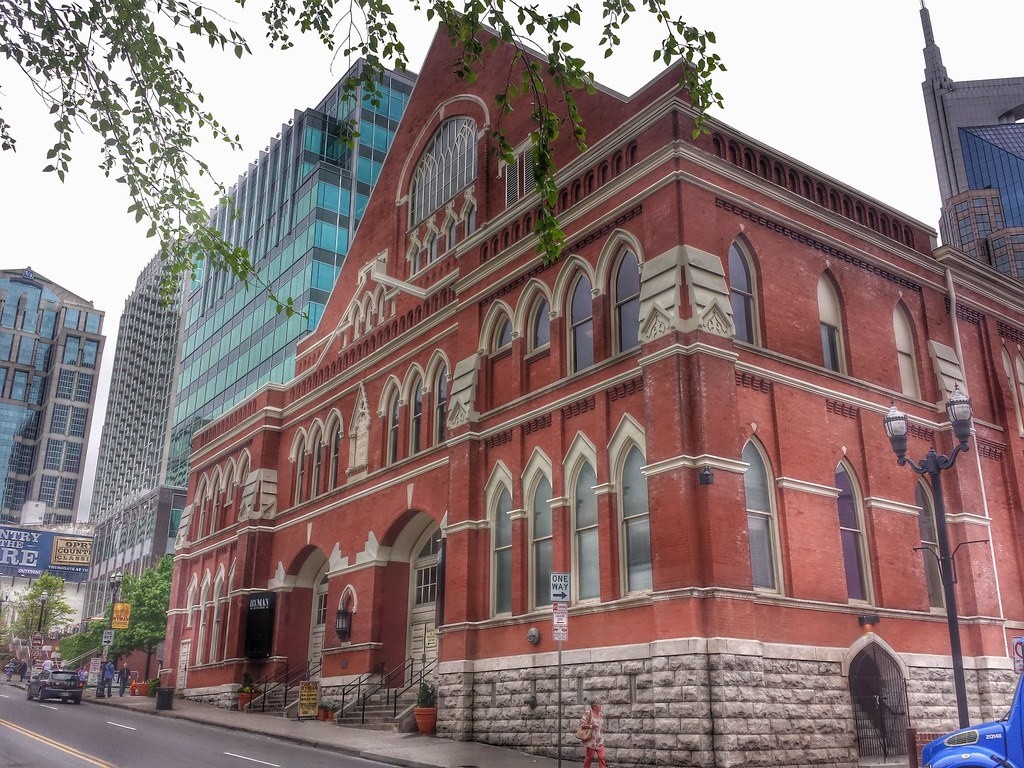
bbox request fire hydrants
[130,680,136,696]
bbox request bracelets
[590,725,592,728]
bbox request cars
[25,668,83,705]
[920,638,1023,768]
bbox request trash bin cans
[155,686,175,710]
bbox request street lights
[881,377,994,733]
[38,588,50,632]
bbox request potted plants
[318,704,328,718]
[327,706,338,720]
[413,679,437,735]
[237,672,259,711]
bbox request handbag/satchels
[575,710,594,740]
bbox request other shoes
[9,679,11,681]
[120,693,123,697]
[103,694,105,698]
[20,680,23,682]
[6,679,8,681]
[108,694,112,697]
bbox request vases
[137,683,149,696]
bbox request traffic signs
[550,572,571,602]
[102,630,115,646]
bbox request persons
[104,659,115,697]
[580,698,606,768]
[18,659,28,682]
[42,658,54,671]
[78,665,87,683]
[117,662,131,696]
[5,658,15,681]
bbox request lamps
[335,608,351,642]
[700,466,714,485]
